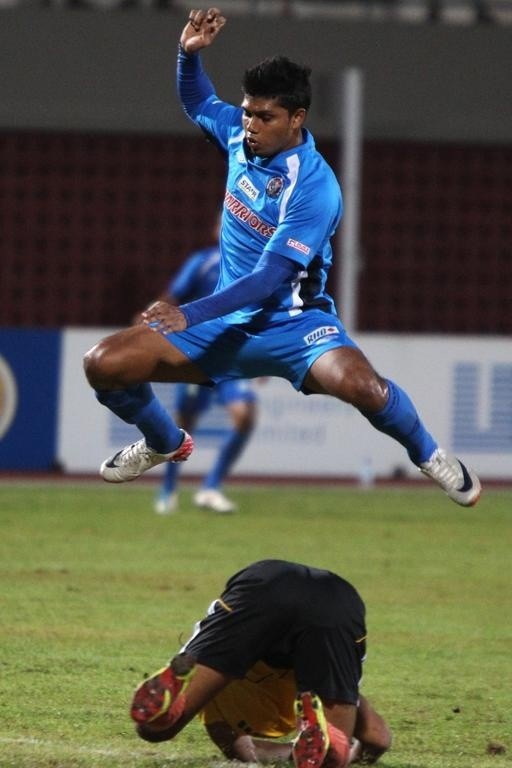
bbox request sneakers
[291,689,331,768]
[154,487,235,514]
[99,428,195,483]
[130,652,198,726]
[407,444,482,507]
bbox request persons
[131,559,392,768]
[84,8,482,506]
[133,210,267,516]
